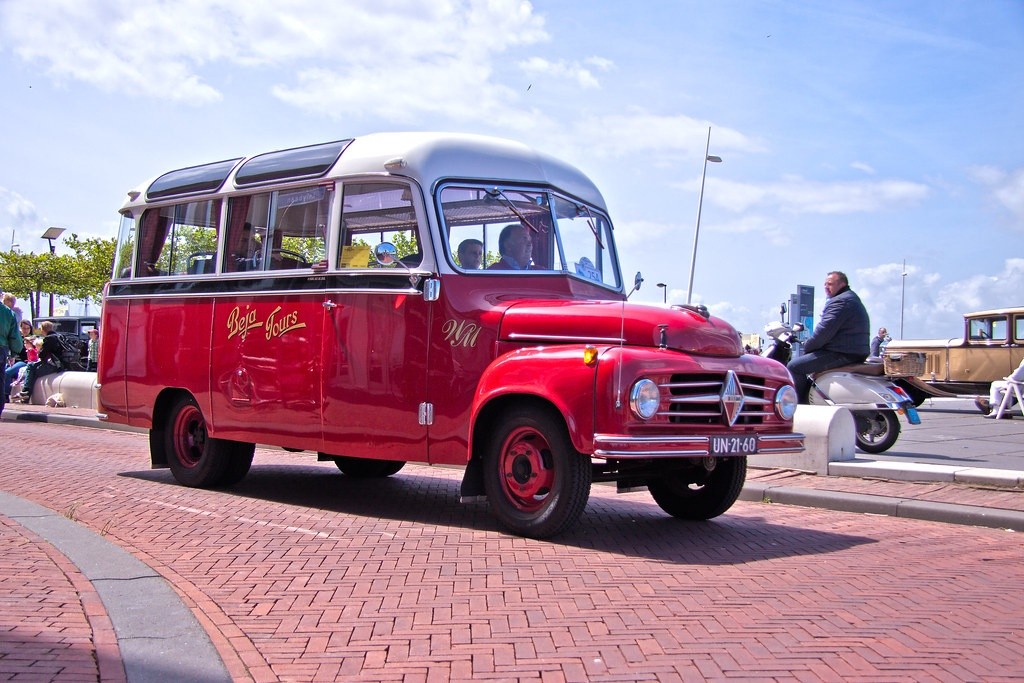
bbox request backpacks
[44,332,81,370]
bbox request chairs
[996,376,1024,420]
[185,251,216,274]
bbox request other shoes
[5,395,10,403]
[11,390,30,404]
[1002,414,1014,419]
[983,413,998,419]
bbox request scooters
[755,301,922,454]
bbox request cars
[31,316,100,356]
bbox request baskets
[884,351,927,378]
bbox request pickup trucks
[879,309,1023,411]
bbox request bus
[95,126,807,542]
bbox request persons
[0,286,99,419]
[786,271,870,405]
[871,328,886,358]
[211,221,549,272]
[983,359,1024,418]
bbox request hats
[87,329,99,338]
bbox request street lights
[899,259,910,340]
[656,281,669,306]
[684,126,722,305]
